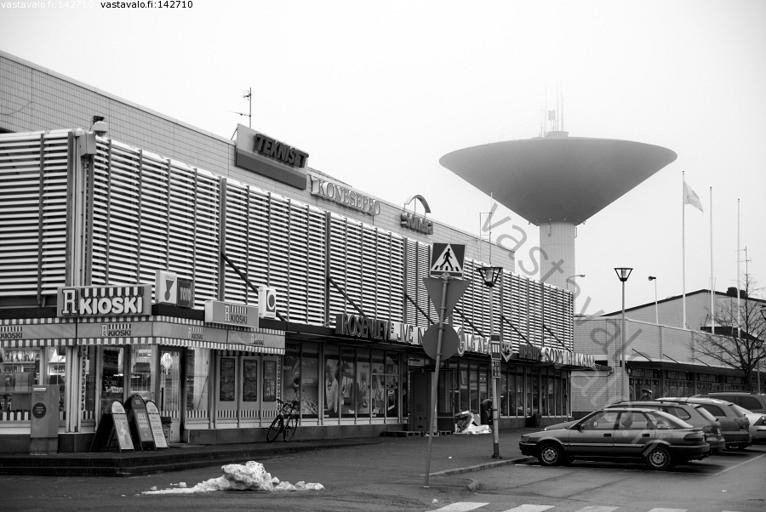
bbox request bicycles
[266,399,299,443]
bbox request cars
[518,391,765,470]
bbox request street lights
[475,266,503,458]
[613,267,632,402]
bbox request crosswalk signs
[430,242,464,274]
